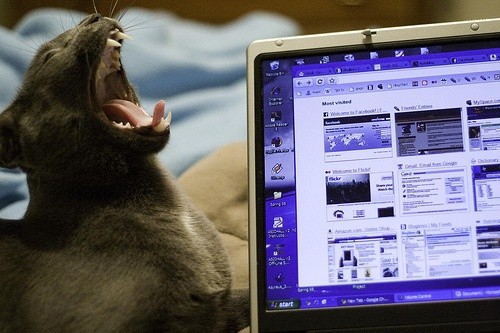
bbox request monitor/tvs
[247,19,497,333]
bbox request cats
[1,1,250,333]
